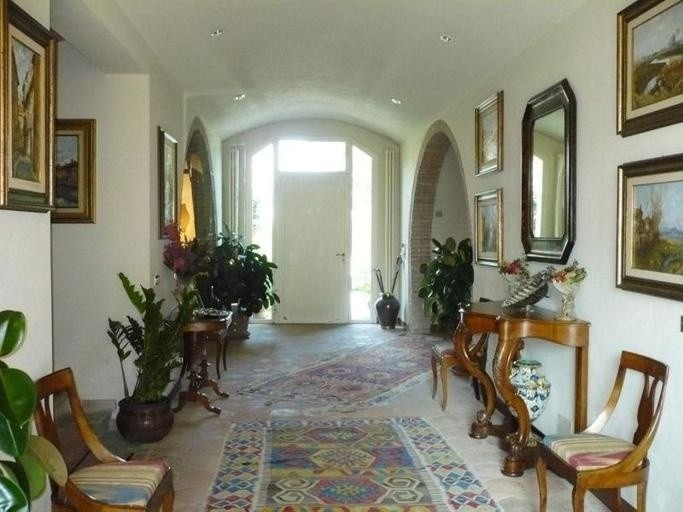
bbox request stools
[179,328,231,381]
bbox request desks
[169,307,234,414]
[451,299,591,476]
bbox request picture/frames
[0,0,57,213]
[616,0,683,137]
[615,152,683,301]
[157,126,178,241]
[473,188,503,268]
[474,90,505,178]
[50,118,96,224]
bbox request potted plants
[202,231,281,339]
[104,271,200,443]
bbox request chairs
[34,366,176,512]
[535,350,670,511]
[431,297,495,412]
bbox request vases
[175,274,198,311]
[556,284,579,322]
[375,293,400,329]
[505,358,551,447]
[507,274,521,292]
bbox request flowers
[497,259,530,278]
[163,220,209,293]
[550,259,587,296]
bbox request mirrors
[521,79,577,265]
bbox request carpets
[199,418,502,511]
[234,333,453,413]
